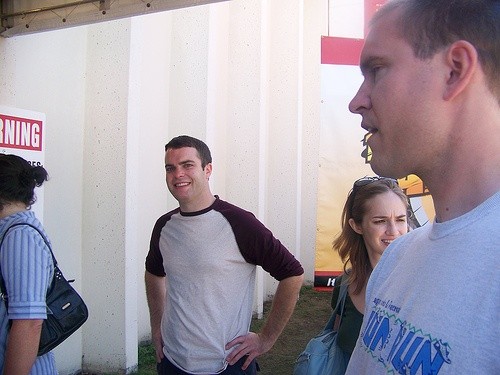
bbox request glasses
[348,175,399,218]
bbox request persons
[340,0,500,375]
[330,176,423,375]
[145,136,304,375]
[0,153,60,375]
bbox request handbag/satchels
[1,223,89,356]
[292,269,350,375]
